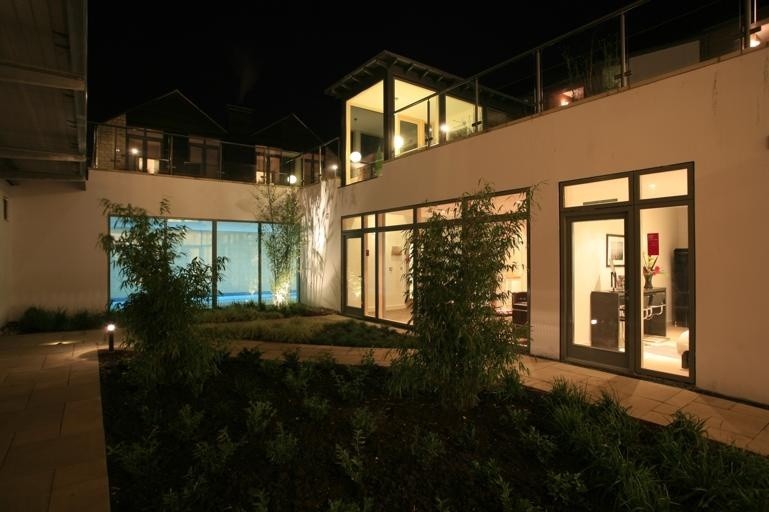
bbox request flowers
[642,255,660,275]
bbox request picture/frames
[605,233,626,267]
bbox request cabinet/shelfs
[589,287,668,352]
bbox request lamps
[105,323,116,352]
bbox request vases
[643,274,653,289]
